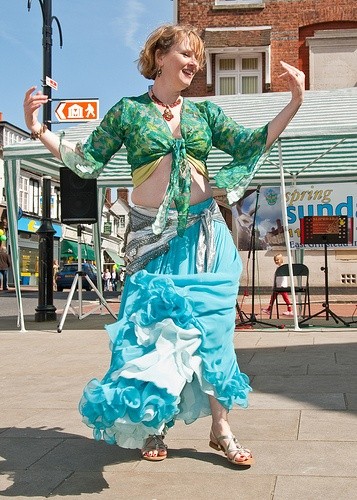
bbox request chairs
[269,263,311,320]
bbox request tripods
[57,224,118,333]
[232,192,284,328]
[296,214,353,327]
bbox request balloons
[0,235,6,241]
[0,229,4,235]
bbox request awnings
[61,239,95,260]
[106,250,126,266]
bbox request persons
[24,24,305,465]
[261,254,293,315]
[0,246,11,290]
[52,259,59,291]
[101,268,126,291]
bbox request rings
[296,73,300,76]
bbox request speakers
[59,166,98,224]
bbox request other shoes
[282,311,293,316]
[262,309,271,315]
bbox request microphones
[256,184,261,191]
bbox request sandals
[208,428,255,466]
[140,435,169,460]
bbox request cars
[55,262,105,292]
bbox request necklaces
[150,88,182,121]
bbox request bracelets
[31,124,47,139]
